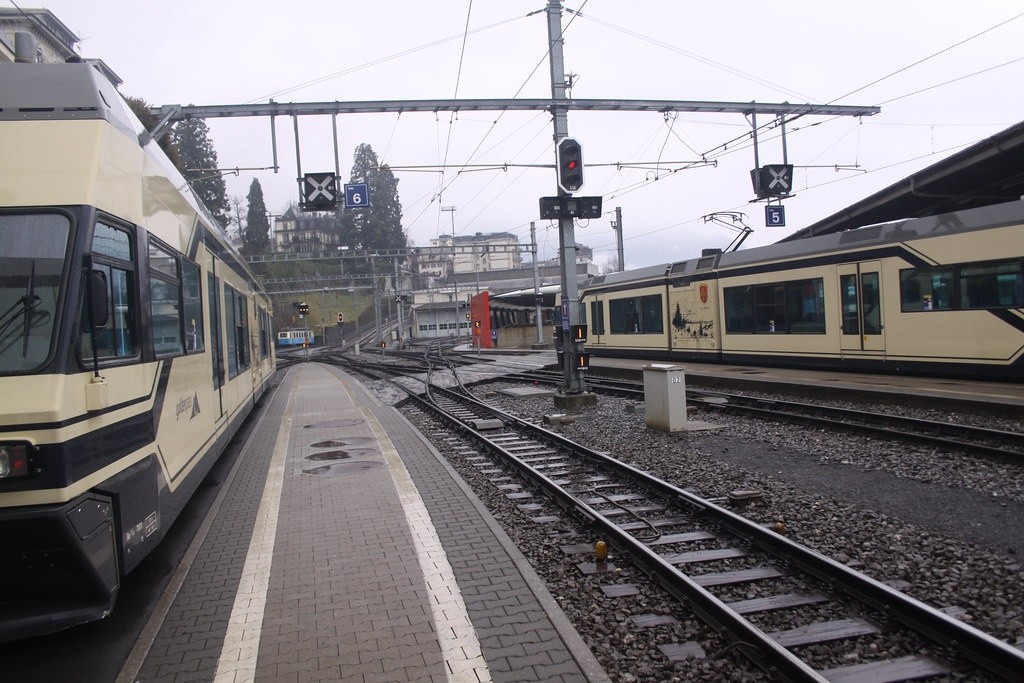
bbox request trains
[554,199,1024,384]
[278,327,314,347]
[0,31,278,647]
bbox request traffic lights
[557,136,584,194]
[338,312,344,323]
